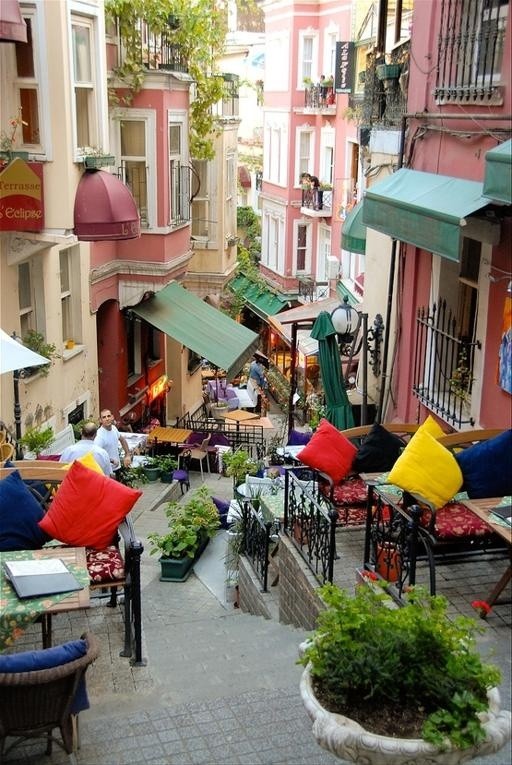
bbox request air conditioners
[325,256,340,281]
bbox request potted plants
[293,579,510,765]
[146,485,222,582]
[142,452,177,484]
[212,403,227,420]
[21,329,57,378]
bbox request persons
[250,358,265,388]
[93,408,132,485]
[59,421,115,478]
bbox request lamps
[330,294,385,377]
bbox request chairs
[178,434,212,481]
[0,633,100,762]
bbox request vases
[2,150,29,163]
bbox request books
[3,556,84,600]
[487,506,512,529]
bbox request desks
[221,409,260,443]
[147,427,191,462]
[459,500,511,618]
[236,445,310,556]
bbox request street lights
[8,331,46,460]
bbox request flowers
[0,106,25,172]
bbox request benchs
[1,461,149,666]
[317,424,512,608]
[233,378,261,413]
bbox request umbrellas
[308,309,362,450]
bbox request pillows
[288,430,312,445]
[0,639,90,716]
[411,413,447,445]
[47,453,108,498]
[298,418,359,486]
